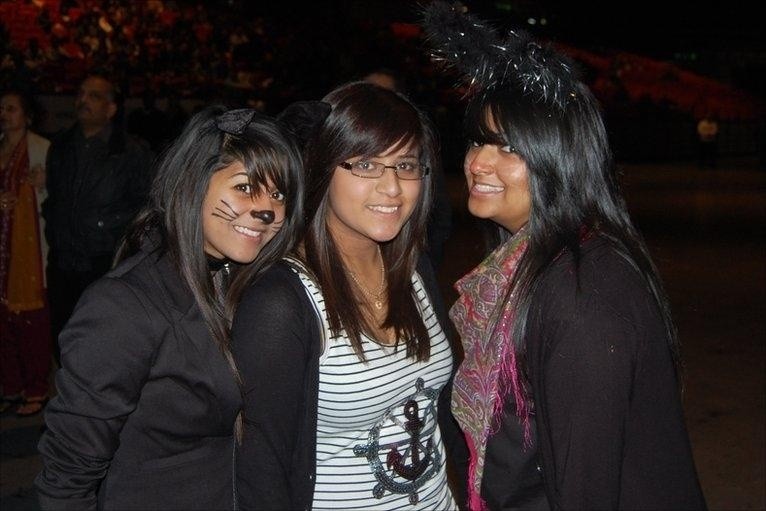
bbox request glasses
[337,160,431,179]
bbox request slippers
[0,394,50,418]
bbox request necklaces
[330,233,391,314]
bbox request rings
[3,204,7,208]
[3,199,8,203]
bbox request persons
[28,98,333,510]
[44,71,162,362]
[0,86,62,419]
[439,69,708,511]
[229,80,473,511]
